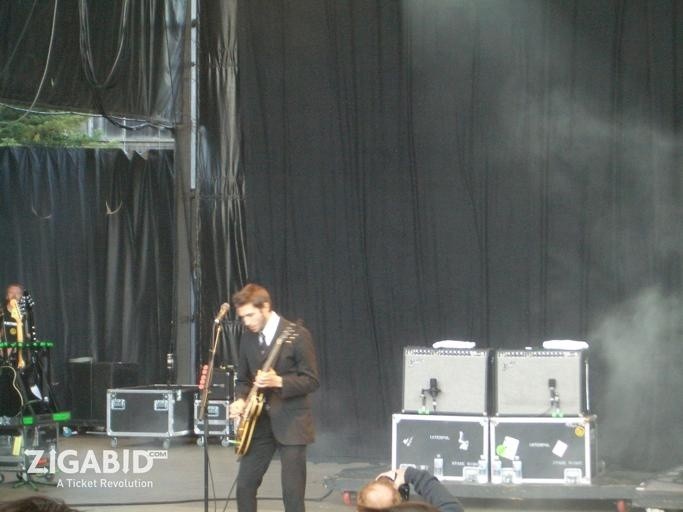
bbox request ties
[257,334,267,359]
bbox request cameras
[376,471,409,501]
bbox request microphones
[212,302,230,325]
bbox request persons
[229,283,321,512]
[0,284,31,359]
[355,466,464,512]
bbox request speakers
[199,368,234,400]
[70,361,137,433]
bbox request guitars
[0,366,25,417]
[0,308,23,418]
[235,324,299,456]
